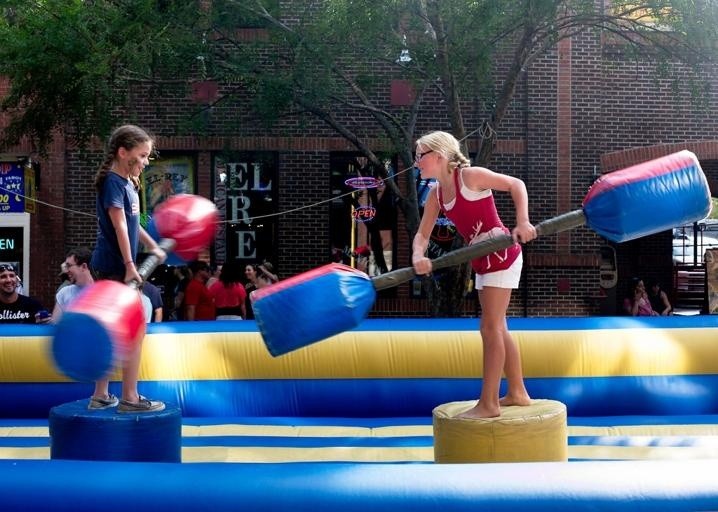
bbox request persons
[0,263,40,325]
[647,284,673,315]
[411,130,539,420]
[135,273,153,321]
[86,124,165,415]
[623,277,655,316]
[169,257,278,321]
[55,262,73,294]
[142,280,164,323]
[36,248,96,324]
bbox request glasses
[415,150,434,159]
[0,264,16,272]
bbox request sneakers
[117,395,166,415]
[87,393,119,410]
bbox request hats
[57,262,68,276]
